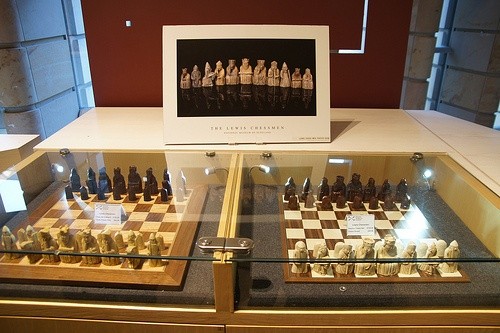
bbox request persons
[401,240,417,275]
[291,240,310,273]
[313,244,331,275]
[180,58,313,89]
[69,166,187,195]
[336,245,356,275]
[442,240,460,273]
[354,237,376,276]
[146,233,163,268]
[376,234,400,276]
[1,224,145,270]
[283,173,411,211]
[424,240,440,275]
